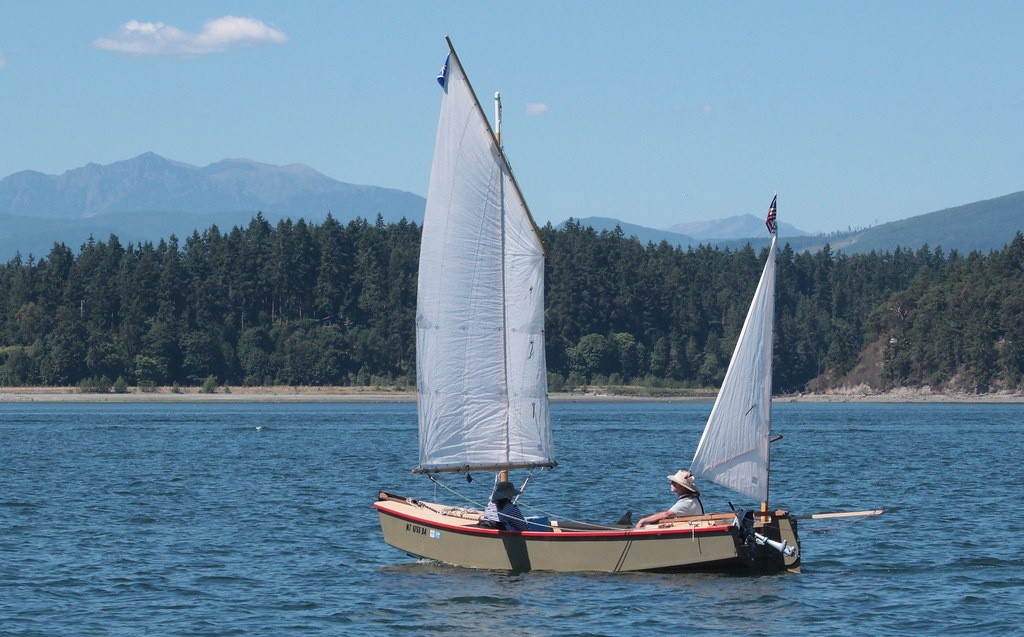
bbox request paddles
[660,510,787,517]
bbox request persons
[636,469,704,528]
[484,481,531,531]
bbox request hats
[667,470,696,492]
[492,482,521,499]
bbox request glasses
[671,481,676,484]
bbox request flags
[766,193,776,234]
[437,54,450,94]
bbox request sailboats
[372,35,883,574]
